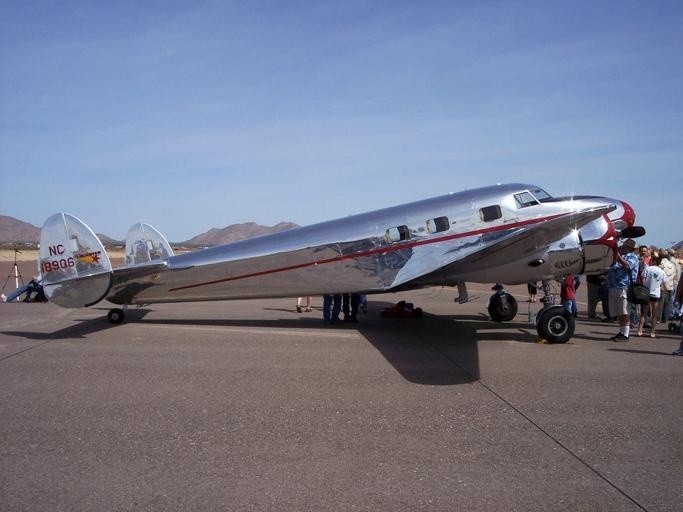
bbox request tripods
[0,254,28,302]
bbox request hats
[621,239,639,249]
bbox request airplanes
[40,183,646,345]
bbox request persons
[342,293,360,323]
[527,282,538,304]
[323,294,342,325]
[296,297,314,313]
[585,238,683,357]
[572,275,581,318]
[359,293,368,313]
[559,274,576,314]
[20,272,48,303]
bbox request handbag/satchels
[627,284,650,305]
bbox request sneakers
[296,305,312,312]
[673,349,683,356]
[611,332,629,342]
[324,316,358,326]
[631,319,683,338]
[588,315,617,323]
